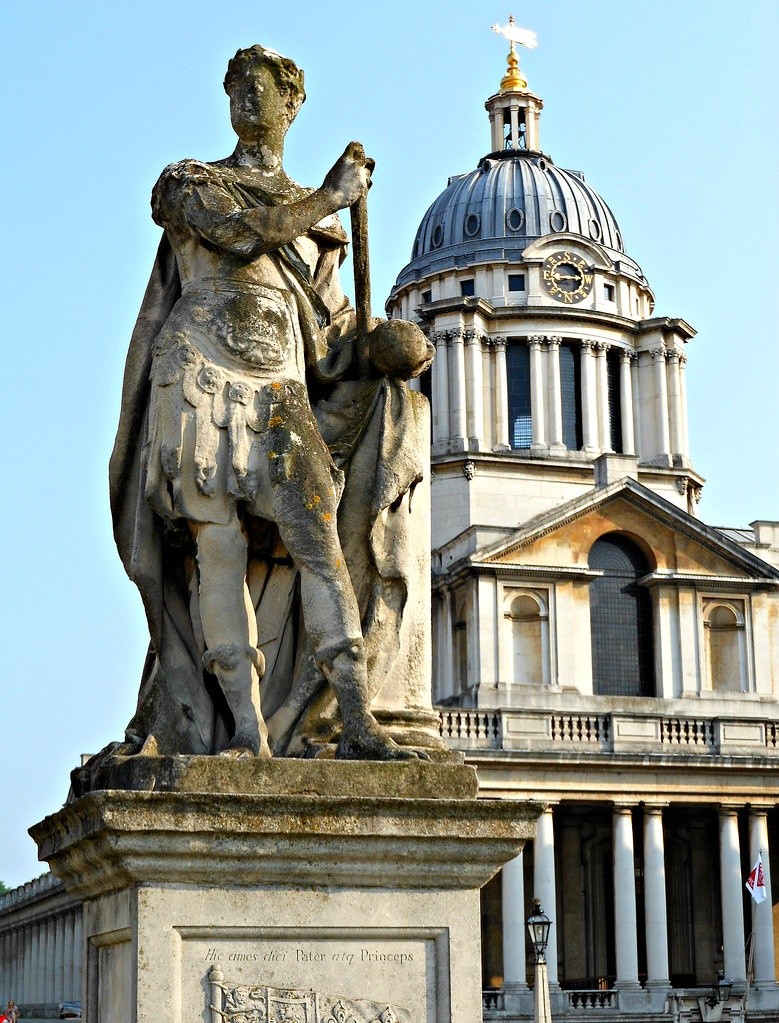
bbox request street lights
[523,897,554,1023]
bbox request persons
[150,47,432,761]
[0,999,19,1023]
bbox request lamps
[705,974,735,1009]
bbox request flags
[744,855,770,903]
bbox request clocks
[541,251,594,305]
